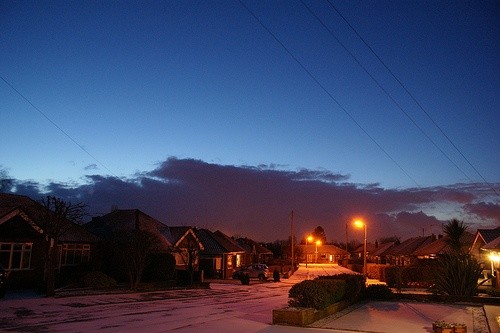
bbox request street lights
[353,220,367,275]
[306,235,313,267]
[315,241,322,265]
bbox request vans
[234,263,270,280]
